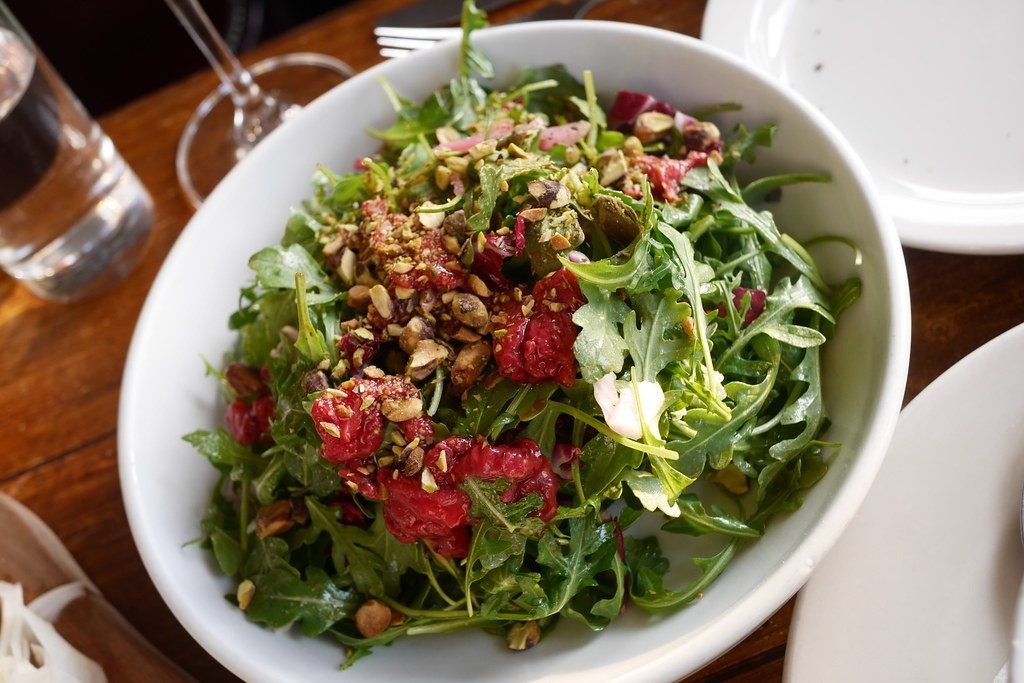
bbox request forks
[371,0,610,57]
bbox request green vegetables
[183,1,860,668]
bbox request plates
[694,0,1024,255]
[782,320,1024,683]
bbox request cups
[0,0,156,305]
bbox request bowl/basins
[117,19,911,683]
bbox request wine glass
[169,0,359,212]
